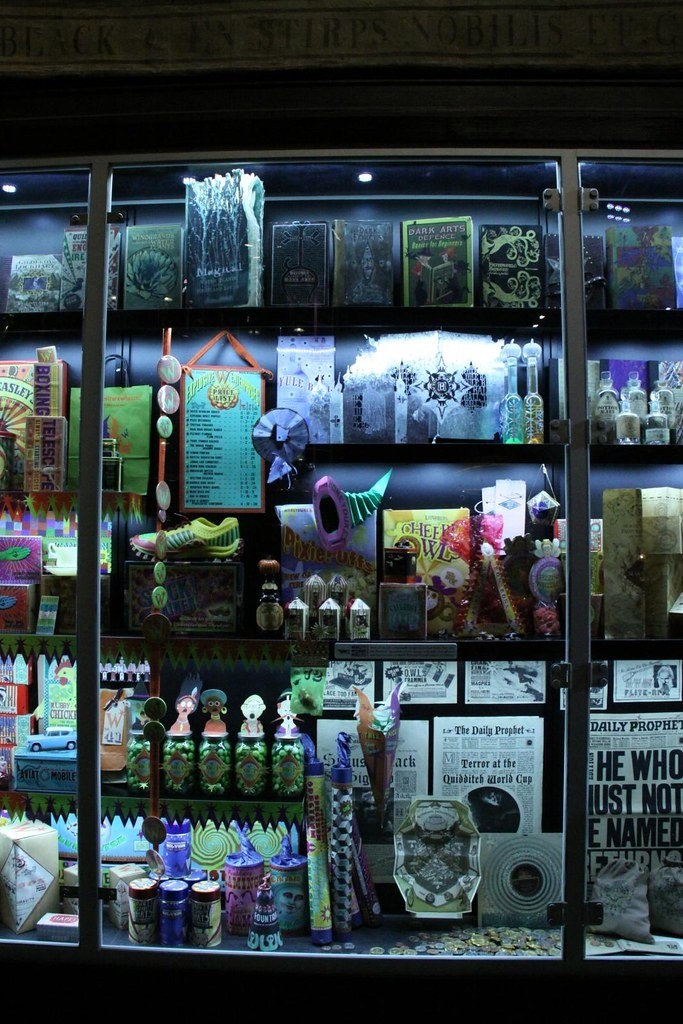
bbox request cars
[25,727,77,752]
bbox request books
[6,166,683,314]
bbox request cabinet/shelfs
[0,148,683,978]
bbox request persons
[657,665,675,694]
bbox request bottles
[591,371,674,445]
[524,356,544,443]
[500,356,523,444]
[261,572,279,637]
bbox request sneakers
[130,516,243,560]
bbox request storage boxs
[103,438,119,458]
[102,457,123,492]
[109,863,147,929]
[36,913,78,943]
[0,584,36,634]
[63,864,79,915]
[0,360,67,491]
[384,547,418,582]
[13,747,77,793]
[0,820,61,935]
[379,582,427,640]
